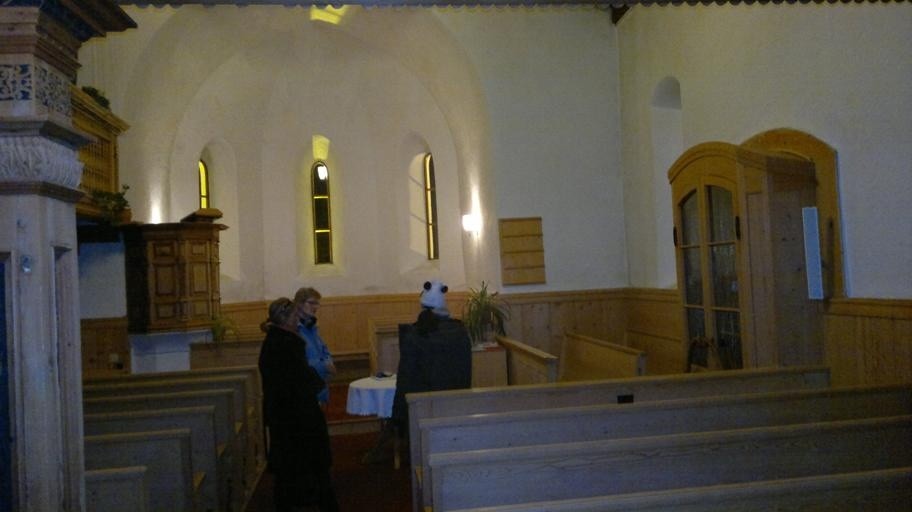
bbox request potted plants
[460,280,512,346]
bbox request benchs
[496,325,683,385]
[84,365,261,512]
[405,365,911,512]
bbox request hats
[419,280,448,310]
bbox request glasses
[305,301,320,305]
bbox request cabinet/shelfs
[122,207,229,335]
[72,84,133,225]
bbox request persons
[391,281,472,476]
[238,298,324,511]
[293,286,336,472]
